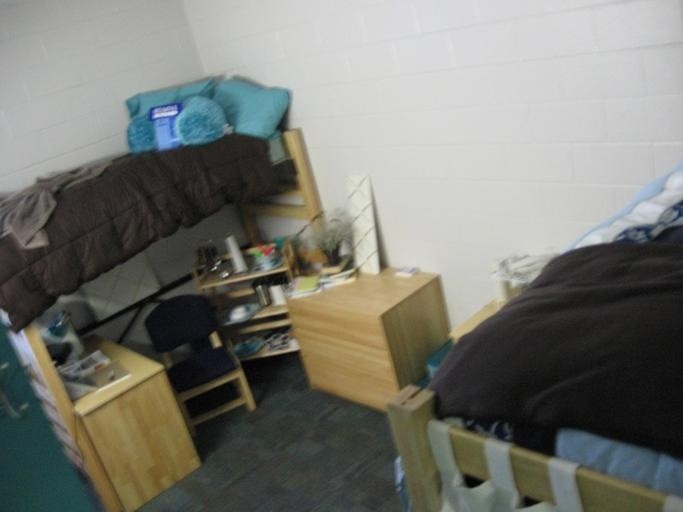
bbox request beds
[388,166,683,512]
[0,128,326,512]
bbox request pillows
[124,76,216,120]
[212,78,290,140]
[127,95,234,153]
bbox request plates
[237,335,262,356]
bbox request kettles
[252,277,270,306]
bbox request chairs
[145,295,258,437]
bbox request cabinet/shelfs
[52,334,203,512]
[286,270,452,413]
[191,239,302,361]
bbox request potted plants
[297,210,356,265]
